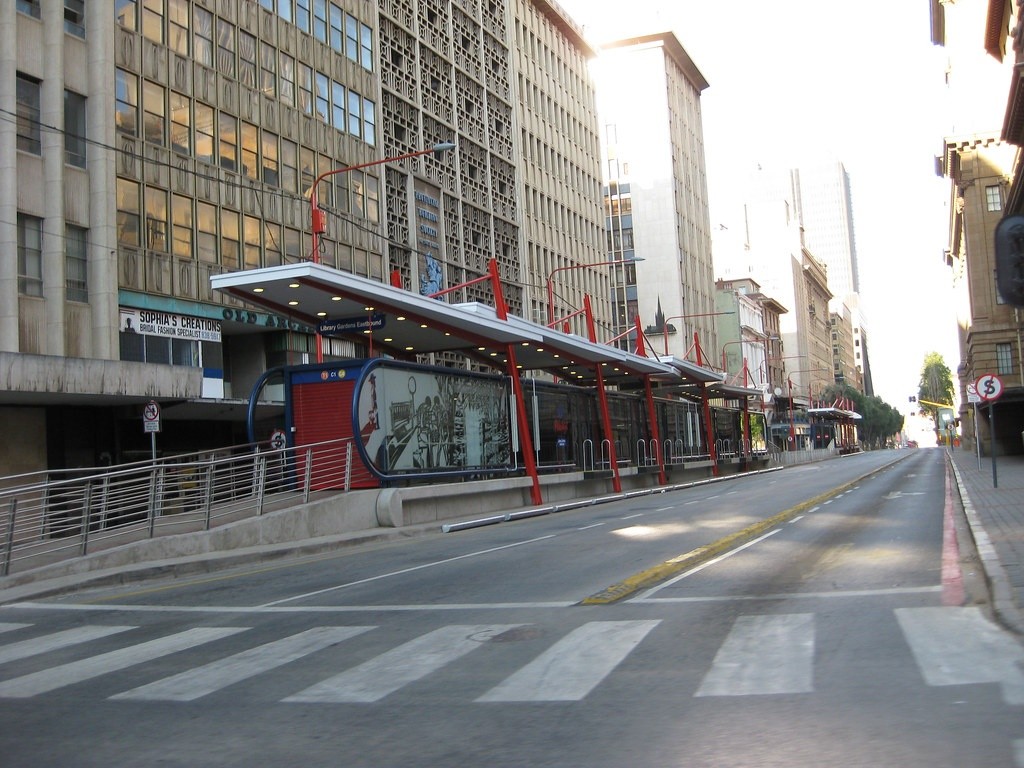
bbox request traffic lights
[909,396,912,402]
[913,396,916,402]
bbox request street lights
[787,367,828,450]
[547,256,645,379]
[721,338,779,406]
[664,312,737,357]
[307,142,459,357]
[759,354,806,385]
